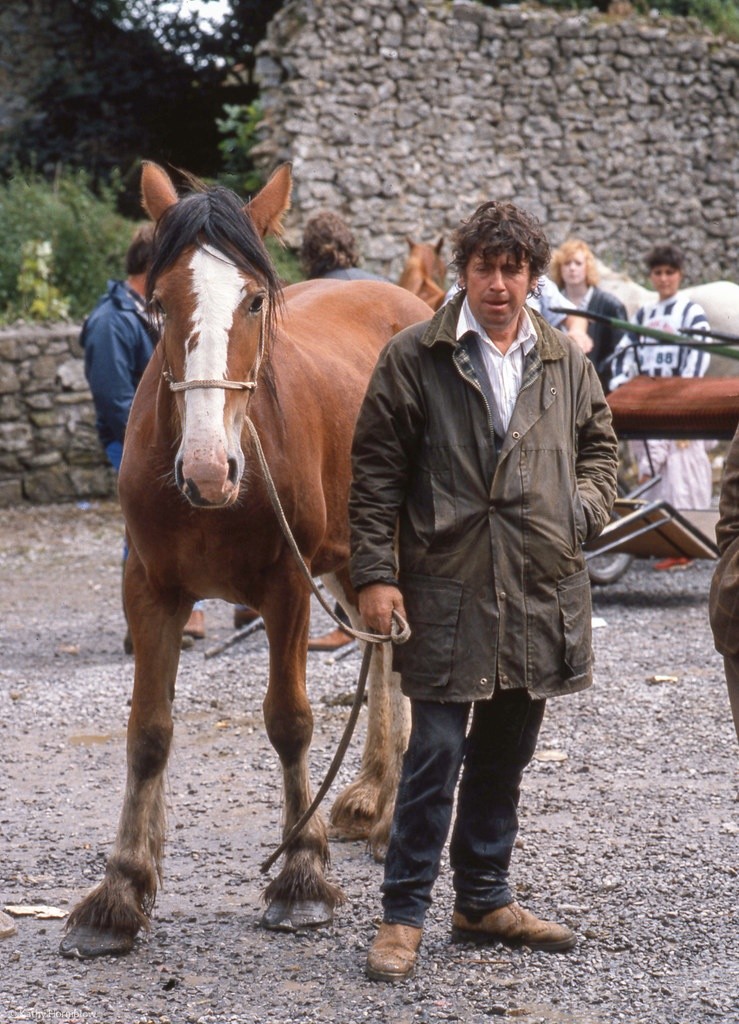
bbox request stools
[602,371,739,444]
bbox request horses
[592,254,738,380]
[397,233,450,311]
[59,156,441,959]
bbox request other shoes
[365,921,422,981]
[452,903,578,952]
[654,556,694,570]
[123,607,355,656]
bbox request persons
[347,201,622,979]
[78,220,194,652]
[607,246,711,571]
[549,239,627,395]
[300,212,386,280]
[524,271,594,351]
